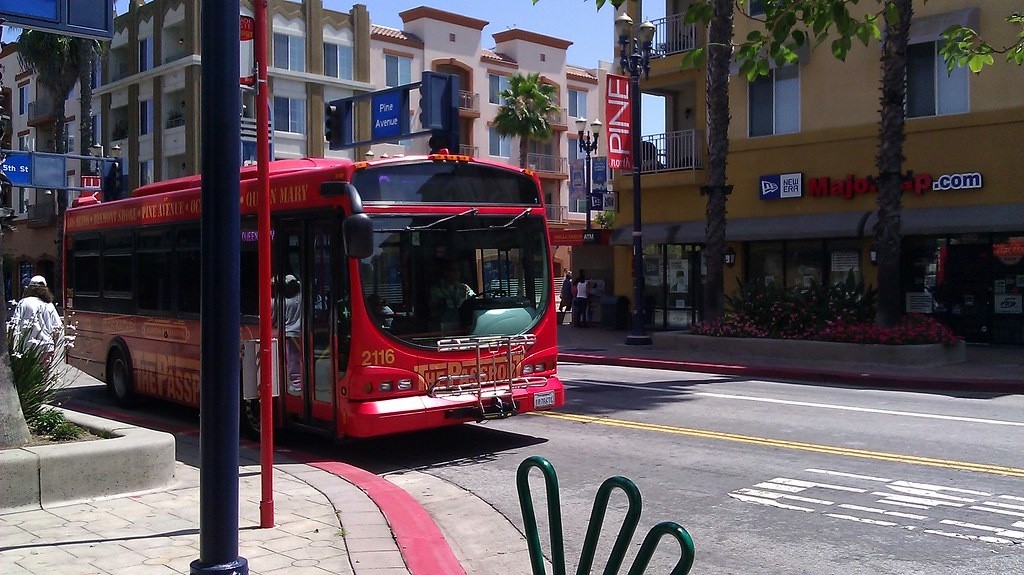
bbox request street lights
[576,116,602,229]
[615,12,656,346]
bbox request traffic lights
[325,106,339,142]
[419,84,423,124]
[109,162,120,189]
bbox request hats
[29,274,47,287]
[284,275,297,285]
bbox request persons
[641,141,665,173]
[10,275,65,401]
[559,272,573,312]
[424,256,478,330]
[367,292,396,329]
[570,278,582,324]
[574,275,589,326]
[21,272,30,291]
[271,274,309,392]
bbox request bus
[63,148,565,454]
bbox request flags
[239,7,258,87]
[604,73,635,171]
